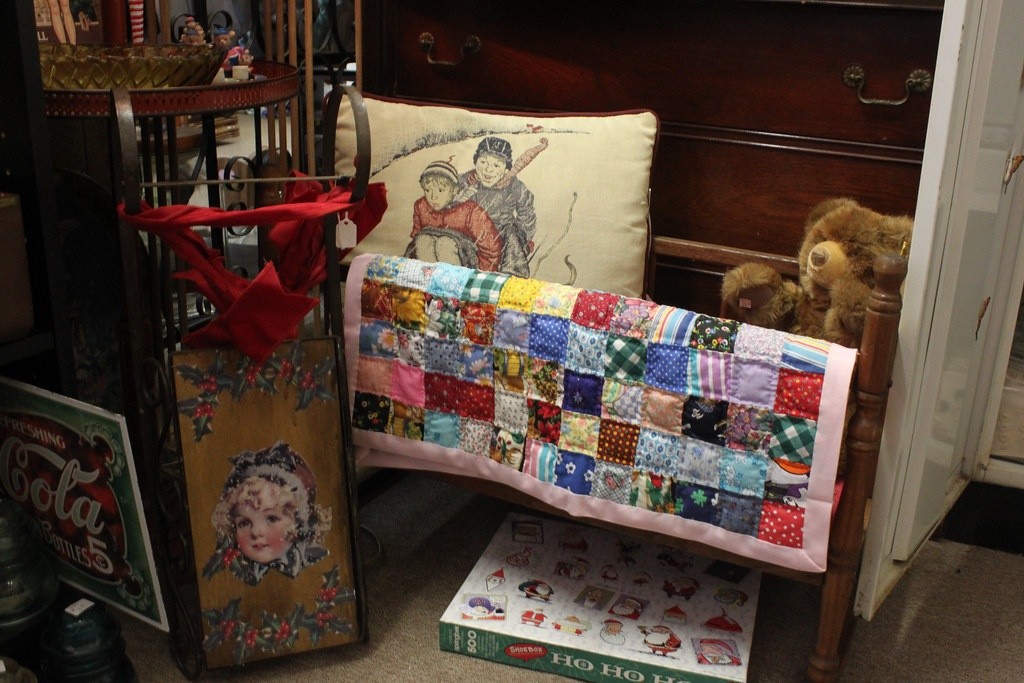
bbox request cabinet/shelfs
[362,0,948,281]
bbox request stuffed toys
[720,197,914,350]
[181,16,256,80]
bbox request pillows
[335,92,657,300]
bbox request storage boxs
[439,511,761,683]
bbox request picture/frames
[168,332,369,670]
[0,376,170,632]
